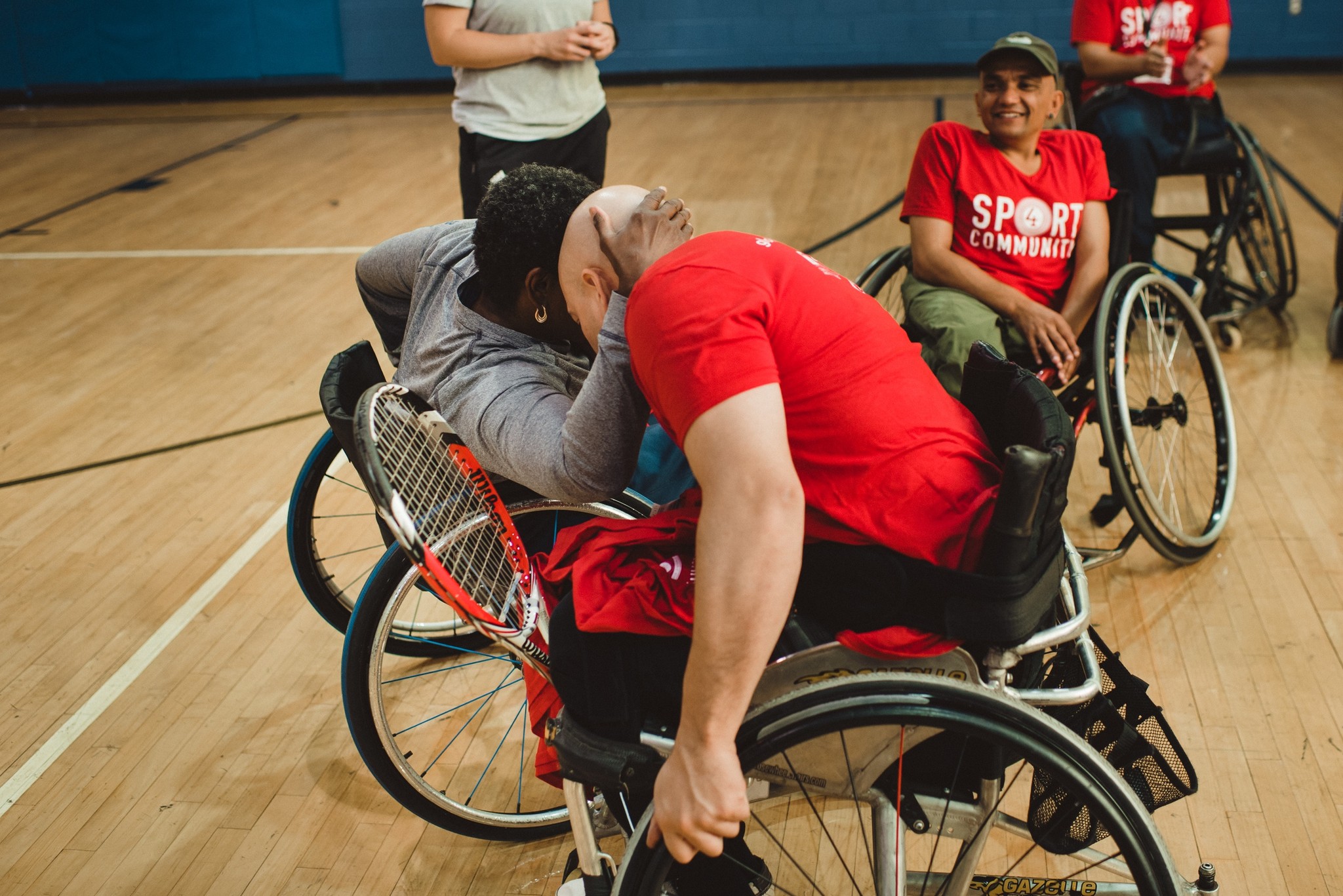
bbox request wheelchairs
[287,340,1216,896]
[853,245,1240,571]
[1054,71,1300,351]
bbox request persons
[557,184,1007,895]
[353,163,694,507]
[422,0,621,221]
[1070,0,1236,271]
[898,31,1117,401]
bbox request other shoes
[659,820,773,896]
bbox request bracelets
[601,21,620,50]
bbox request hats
[976,31,1060,85]
